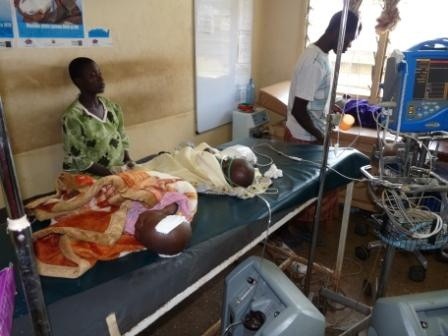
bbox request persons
[284,10,362,246]
[60,57,136,177]
[25,170,198,278]
[335,94,388,130]
[136,142,272,199]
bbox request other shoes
[295,223,327,245]
[277,227,300,246]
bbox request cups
[339,114,355,130]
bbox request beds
[256,80,448,213]
[0,136,371,336]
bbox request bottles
[245,78,256,104]
